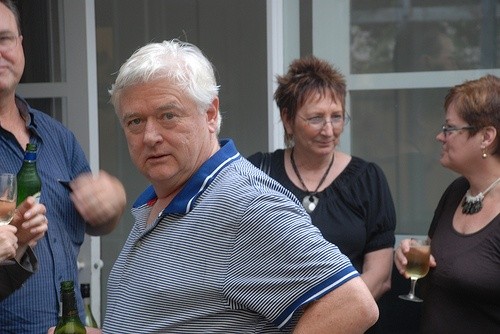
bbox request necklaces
[291,145,335,214]
[462,178,500,215]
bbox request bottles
[10,144,42,207]
[54,279,87,334]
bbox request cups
[0,172,17,225]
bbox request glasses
[296,111,352,126]
[442,125,485,136]
[0,32,20,51]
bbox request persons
[0,195,48,301]
[0,1,128,334]
[246,58,396,334]
[47,38,380,334]
[390,74,500,334]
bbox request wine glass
[398,237,431,302]
